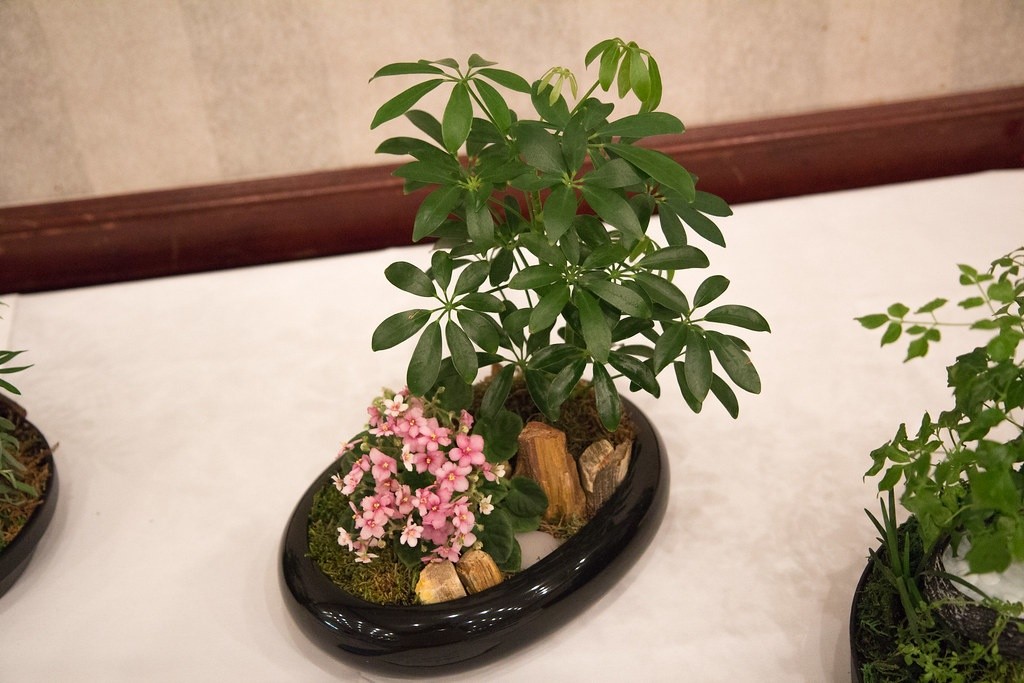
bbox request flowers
[303,36,772,607]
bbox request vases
[277,379,670,679]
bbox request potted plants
[0,298,59,597]
[847,246,1023,683]
